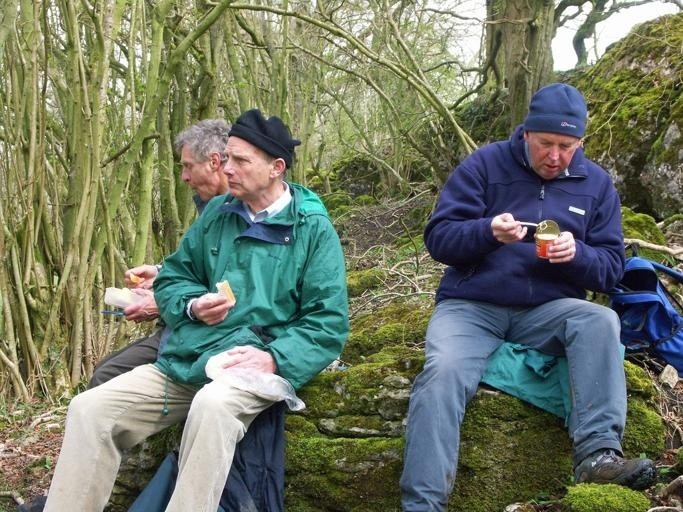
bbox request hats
[228,109,301,169]
[524,83,586,138]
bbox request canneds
[534,220,559,260]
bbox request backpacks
[608,243,682,378]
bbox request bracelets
[189,302,198,321]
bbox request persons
[40,106,351,512]
[398,82,658,511]
[87,116,234,389]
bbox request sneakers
[575,450,657,492]
